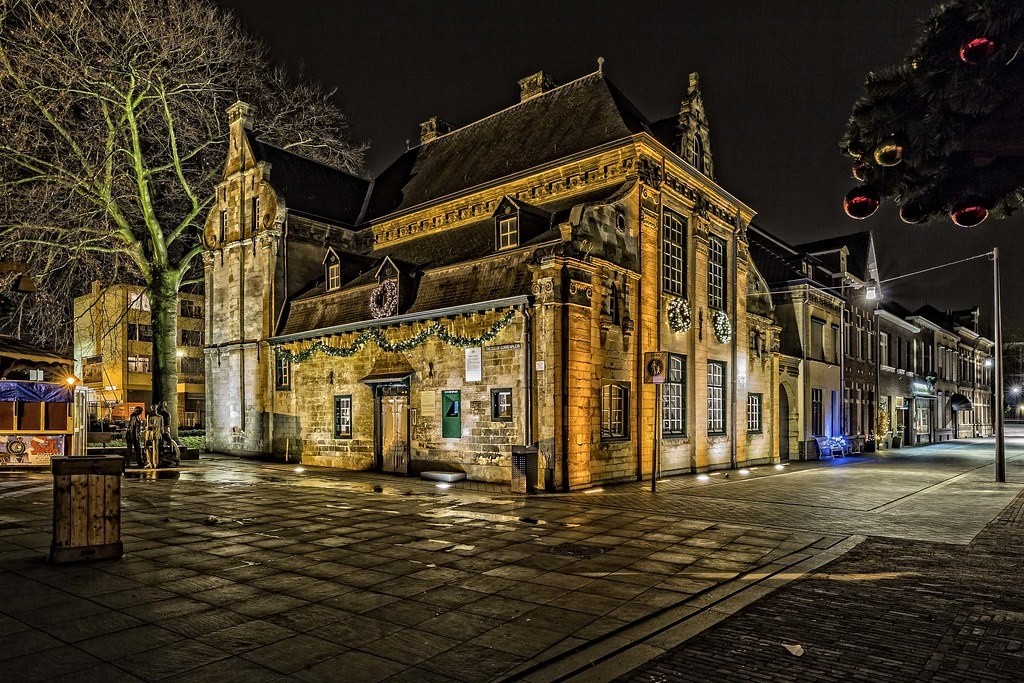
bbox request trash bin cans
[49,454,125,565]
[511,444,537,493]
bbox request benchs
[812,435,847,461]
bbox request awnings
[951,393,974,411]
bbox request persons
[125,406,145,468]
[140,401,181,468]
